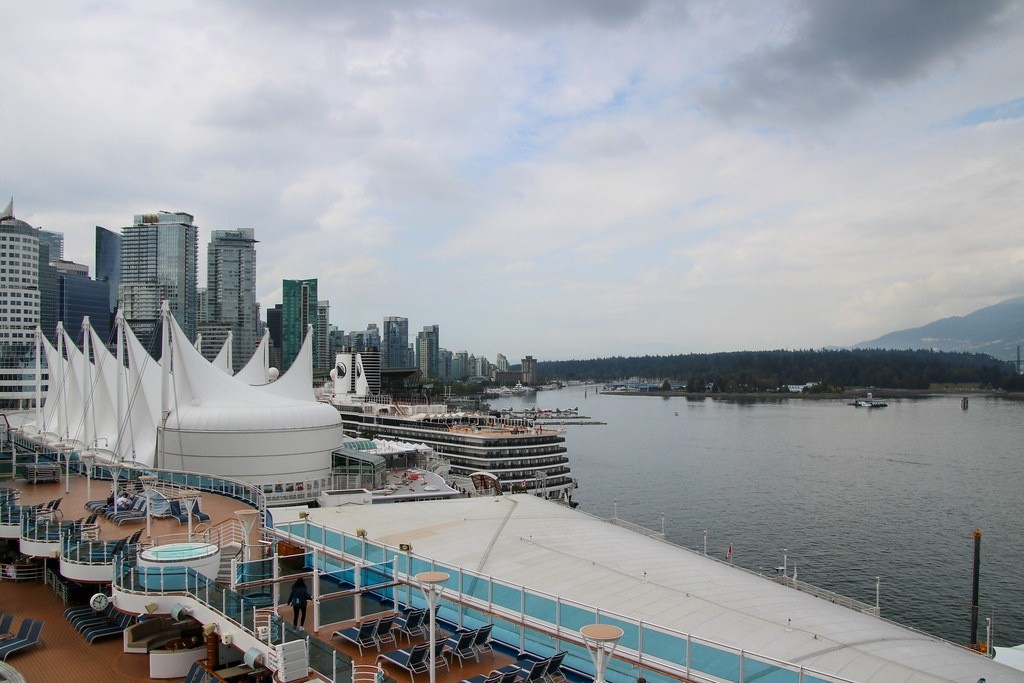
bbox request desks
[164,639,193,649]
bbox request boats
[488,381,526,395]
[316,345,580,509]
[847,399,888,407]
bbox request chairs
[0,460,569,683]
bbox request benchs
[123,615,182,654]
[149,644,242,679]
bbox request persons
[102,493,135,513]
[288,577,313,632]
